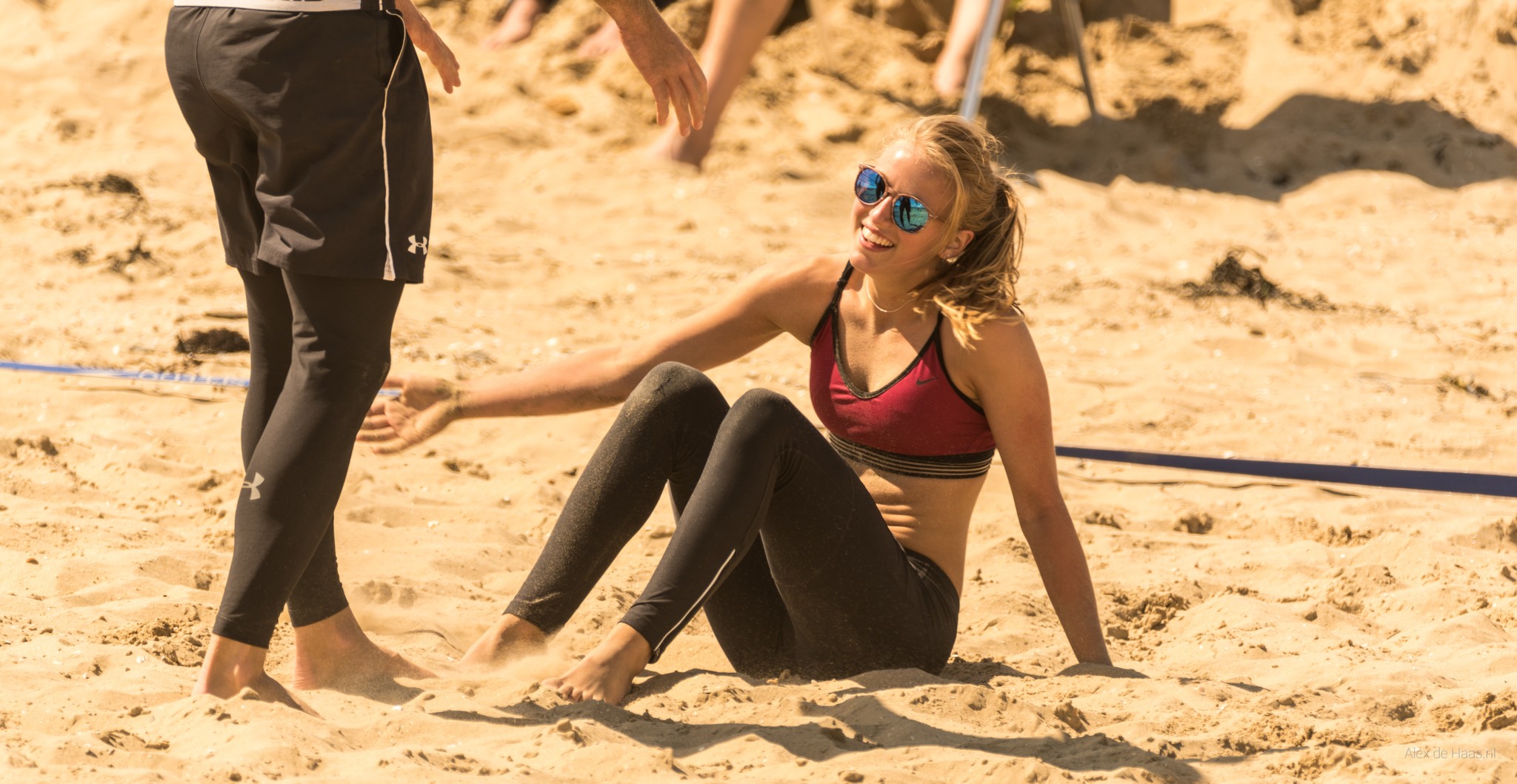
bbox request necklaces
[865,277,919,313]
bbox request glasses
[853,162,966,235]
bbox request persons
[358,117,1116,709]
[166,0,711,715]
[652,0,1005,165]
